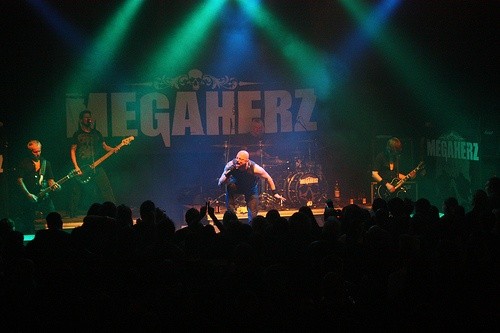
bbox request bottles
[215,201,220,213]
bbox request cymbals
[210,144,272,148]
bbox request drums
[270,173,324,208]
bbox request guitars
[21,170,80,210]
[76,135,135,184]
[377,160,427,201]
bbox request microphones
[224,163,237,177]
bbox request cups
[335,191,340,197]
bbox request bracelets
[271,189,278,195]
[380,179,387,185]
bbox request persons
[218,150,287,226]
[0,176,500,333]
[16,140,61,235]
[372,138,417,202]
[228,121,282,171]
[70,110,121,222]
